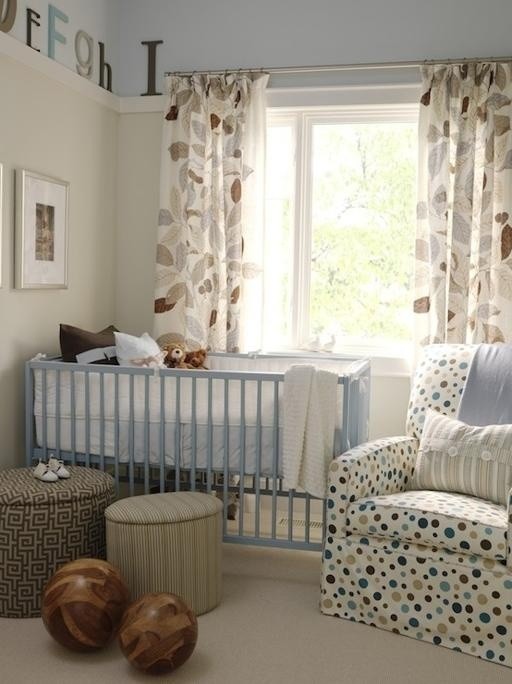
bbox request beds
[24,348,378,555]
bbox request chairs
[322,343,511,669]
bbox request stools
[1,465,226,623]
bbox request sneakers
[48,456,71,477]
[33,462,58,481]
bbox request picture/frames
[12,169,73,292]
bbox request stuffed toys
[181,346,210,370]
[160,342,195,369]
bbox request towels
[275,362,344,501]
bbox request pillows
[413,404,510,496]
[60,326,168,369]
